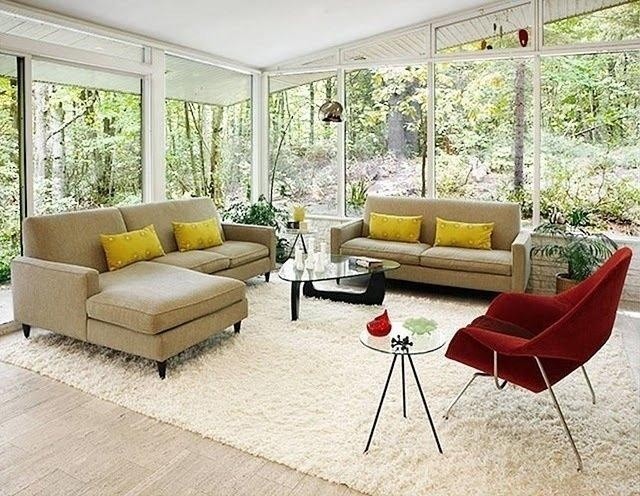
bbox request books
[349,254,382,267]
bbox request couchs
[330,195,532,295]
[9,196,278,381]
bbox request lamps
[271,99,342,204]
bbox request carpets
[0,269,640,495]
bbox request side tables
[286,229,317,261]
[358,318,447,455]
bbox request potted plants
[530,204,618,294]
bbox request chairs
[442,247,633,472]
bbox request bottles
[295,238,328,273]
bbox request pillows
[170,215,224,252]
[433,217,495,250]
[100,224,166,271]
[368,212,423,243]
